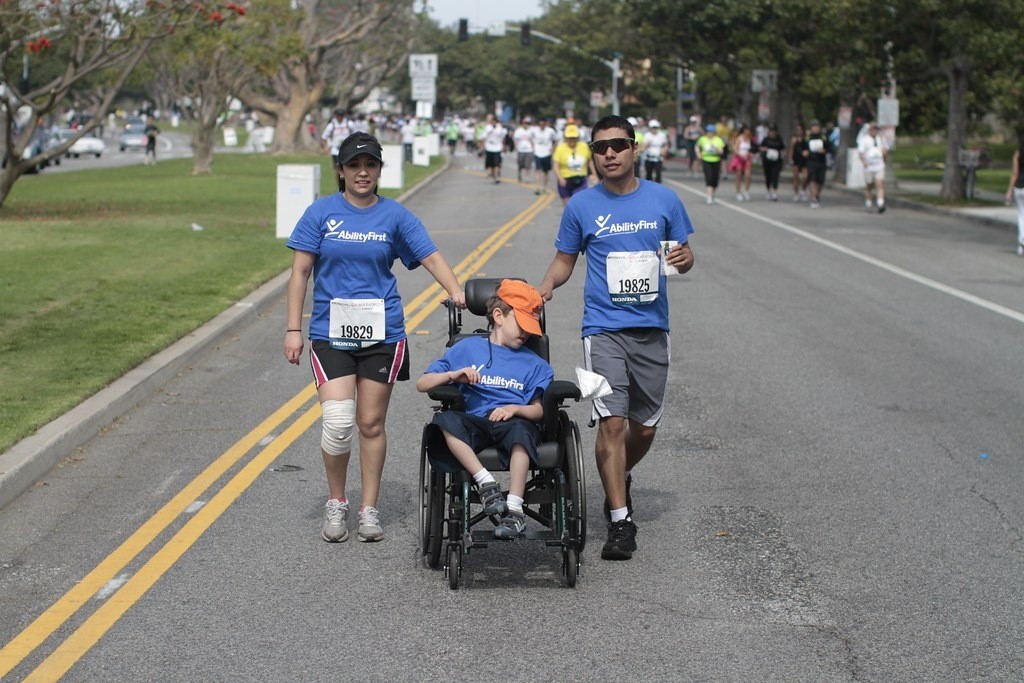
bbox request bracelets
[287,329,301,332]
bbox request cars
[50,129,105,158]
[119,124,149,152]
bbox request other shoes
[706,190,884,211]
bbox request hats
[522,118,530,124]
[648,120,660,128]
[706,124,715,131]
[564,125,578,138]
[770,123,777,129]
[334,109,345,115]
[811,119,820,125]
[869,122,880,129]
[337,141,382,164]
[497,279,543,337]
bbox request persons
[1006,127,1024,255]
[534,115,695,560]
[302,109,594,206]
[858,122,890,212]
[627,116,842,210]
[283,132,468,544]
[418,279,554,540]
[141,115,159,164]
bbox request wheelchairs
[416,277,588,590]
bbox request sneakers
[478,481,507,514]
[603,473,633,521]
[322,495,352,542]
[494,511,525,539]
[600,510,637,558]
[357,506,383,541]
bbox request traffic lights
[520,24,530,45]
[459,19,467,42]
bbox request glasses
[589,137,635,156]
